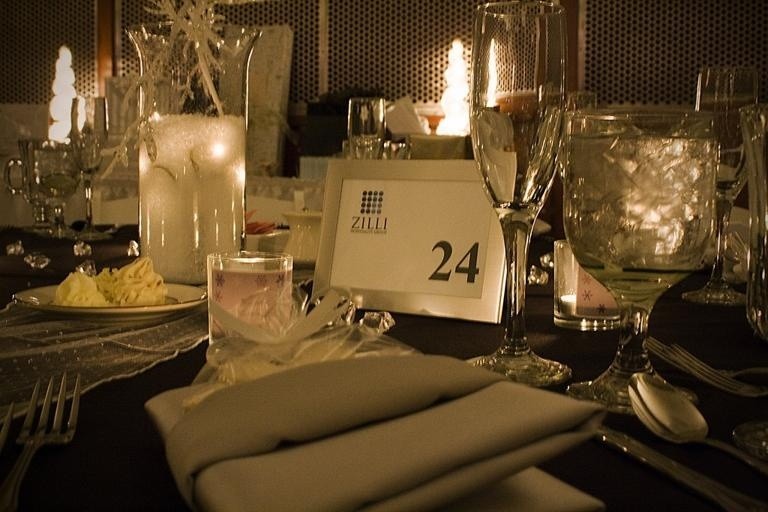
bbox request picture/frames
[310,158,515,325]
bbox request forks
[0,370,84,511]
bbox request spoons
[627,373,767,479]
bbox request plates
[10,273,205,323]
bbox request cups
[551,234,623,331]
[737,103,768,346]
[343,95,411,159]
[207,251,293,360]
[421,110,445,135]
[125,25,264,283]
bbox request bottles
[281,211,322,265]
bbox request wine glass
[464,1,574,391]
[4,128,103,242]
[561,107,719,420]
[693,66,758,310]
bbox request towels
[145,355,606,512]
